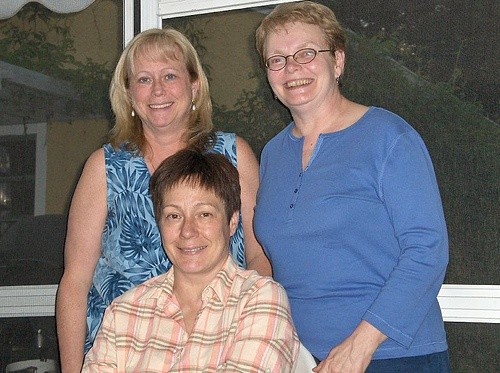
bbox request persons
[252,1,452,373]
[81,150,299,373]
[55,28,272,373]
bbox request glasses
[262,45,339,72]
